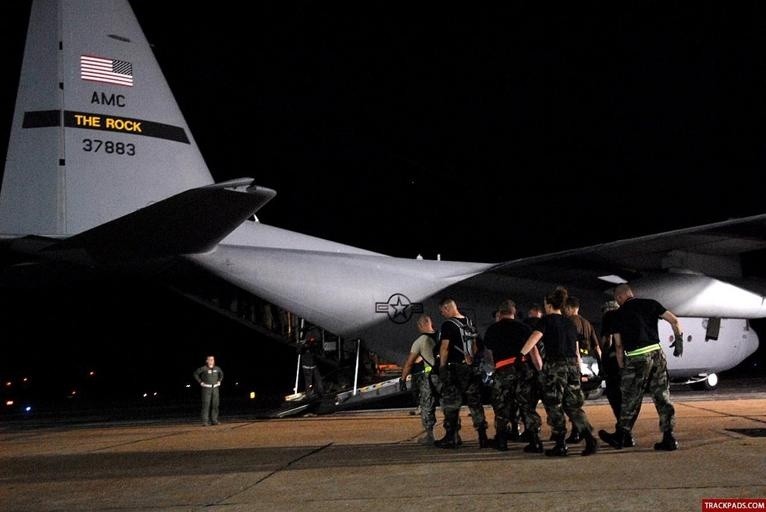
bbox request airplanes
[0,0,766,419]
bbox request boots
[419,429,679,456]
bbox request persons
[398,284,684,456]
[193,356,223,427]
[301,335,328,399]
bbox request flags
[81,55,133,86]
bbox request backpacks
[416,331,441,370]
[443,317,478,368]
